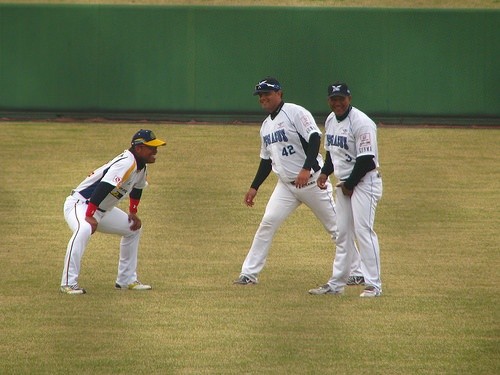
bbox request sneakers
[360,286,381,298]
[346,276,365,285]
[60,284,86,294]
[115,279,151,291]
[231,273,258,284]
[308,285,341,295]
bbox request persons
[234,75,376,287]
[58,130,170,294]
[307,81,390,299]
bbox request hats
[253,77,282,95]
[131,129,166,147]
[327,83,351,99]
